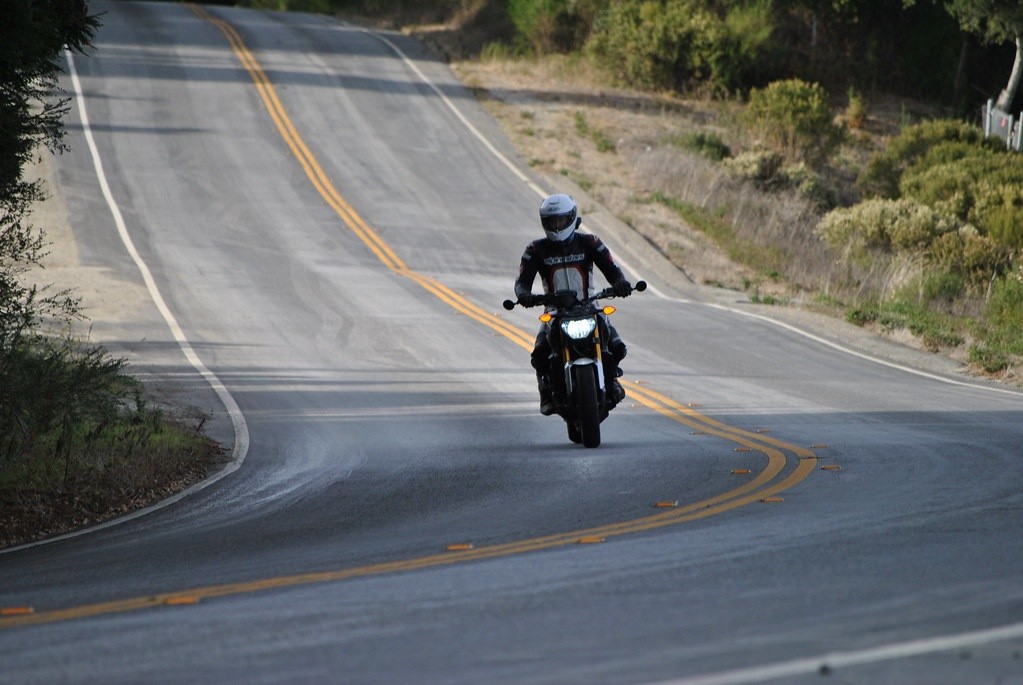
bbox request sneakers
[540,395,554,416]
[611,381,625,403]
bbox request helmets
[538,194,578,241]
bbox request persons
[513,194,628,417]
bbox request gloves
[518,293,537,309]
[613,280,632,297]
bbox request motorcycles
[503,280,649,448]
